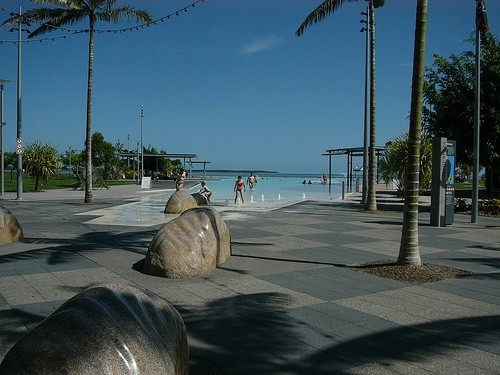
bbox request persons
[198,181,213,203]
[174,168,187,190]
[234,175,246,203]
[247,172,258,190]
